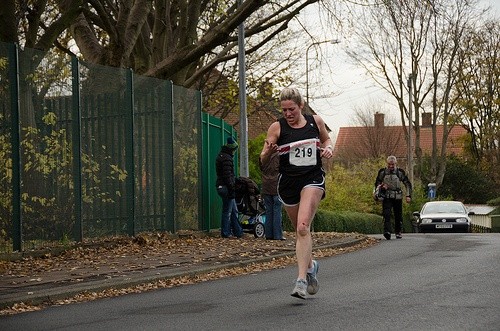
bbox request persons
[375,156,412,240]
[215,136,249,239]
[261,88,333,299]
[259,151,286,241]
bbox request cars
[412,201,474,233]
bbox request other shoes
[266,237,286,241]
[383,232,391,240]
[396,232,402,239]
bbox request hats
[227,136,239,150]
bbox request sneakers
[304,260,320,295]
[290,280,308,300]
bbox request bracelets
[406,195,411,198]
[326,145,333,150]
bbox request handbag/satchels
[373,183,388,201]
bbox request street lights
[305,38,342,104]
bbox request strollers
[234,176,268,239]
